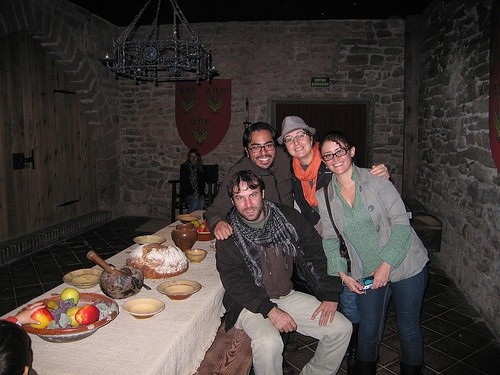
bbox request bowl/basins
[17,293,119,344]
[156,280,202,300]
[186,248,208,264]
[177,214,201,223]
[122,298,166,319]
[62,268,104,290]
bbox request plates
[134,235,167,245]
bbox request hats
[277,116,316,145]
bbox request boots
[400,360,425,375]
[347,323,359,375]
[354,355,377,375]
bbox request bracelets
[341,274,347,287]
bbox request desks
[0,211,253,375]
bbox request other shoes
[285,341,298,352]
[282,360,294,375]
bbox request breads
[125,242,188,279]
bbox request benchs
[195,322,253,375]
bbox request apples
[189,220,209,232]
[29,287,100,329]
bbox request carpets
[135,217,171,232]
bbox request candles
[246,97,250,123]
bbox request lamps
[99,0,218,85]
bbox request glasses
[247,143,276,153]
[322,147,350,162]
[283,132,308,145]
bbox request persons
[315,132,431,375]
[276,116,394,367]
[214,170,353,375]
[204,122,292,240]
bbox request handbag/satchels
[324,184,351,272]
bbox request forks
[195,231,216,242]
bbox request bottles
[355,275,391,291]
[172,224,198,252]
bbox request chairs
[168,164,219,223]
